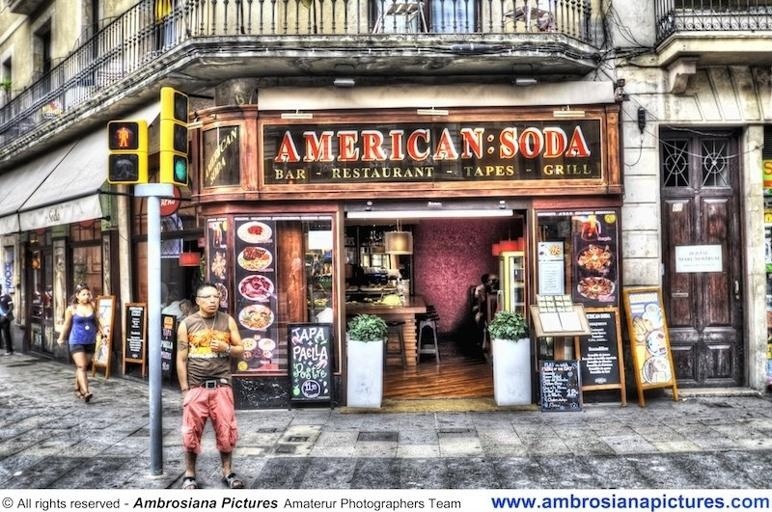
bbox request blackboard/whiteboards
[122,302,147,364]
[161,314,176,377]
[287,323,335,403]
[571,307,624,391]
[539,360,581,413]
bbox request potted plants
[486,310,535,407]
[346,312,390,409]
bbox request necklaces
[200,312,217,343]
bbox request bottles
[213,225,223,249]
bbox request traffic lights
[160,87,189,187]
[107,119,149,184]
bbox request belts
[202,380,218,389]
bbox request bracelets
[181,387,191,393]
[224,343,232,356]
[101,335,107,339]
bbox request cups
[645,303,663,320]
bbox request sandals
[73,385,93,402]
[182,476,199,489]
[221,469,245,489]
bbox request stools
[384,304,441,371]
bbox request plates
[236,221,277,371]
[575,245,614,273]
[214,283,228,303]
[632,310,671,384]
[576,277,615,299]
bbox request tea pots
[581,221,601,242]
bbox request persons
[470,273,499,326]
[175,283,243,490]
[57,282,109,403]
[0,283,14,355]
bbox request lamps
[179,242,202,268]
[417,104,451,117]
[280,106,313,121]
[552,106,586,121]
[490,225,524,257]
[384,220,413,256]
